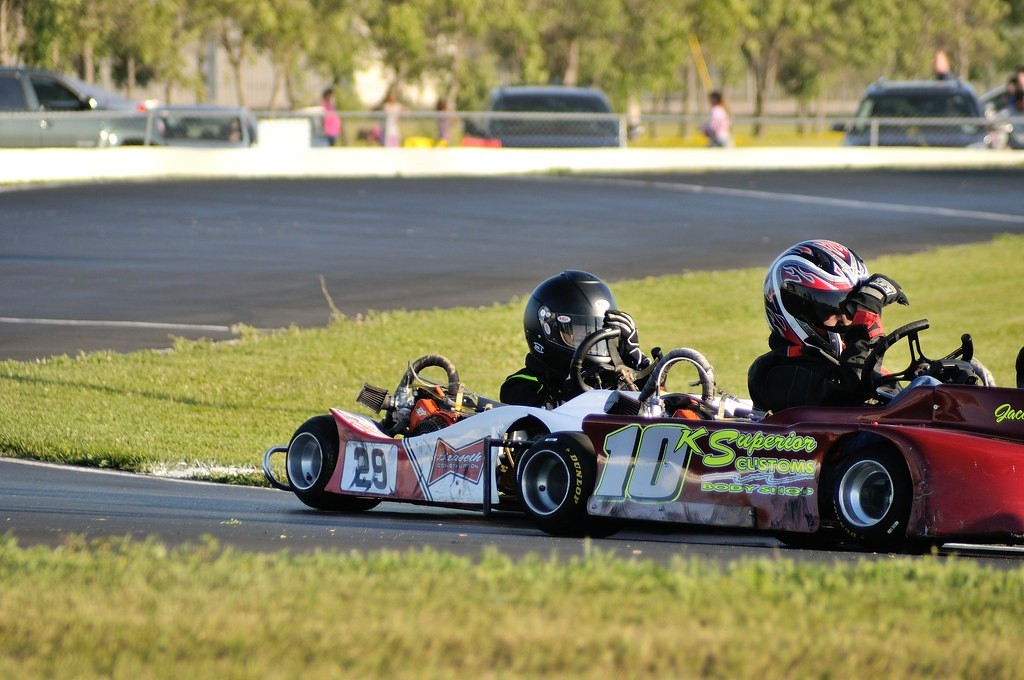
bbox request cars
[0,69,162,148]
[473,86,621,148]
[156,104,257,149]
[981,85,1024,150]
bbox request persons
[320,89,341,146]
[748,239,908,414]
[934,51,1024,114]
[500,270,649,408]
[702,92,732,147]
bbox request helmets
[522,268,619,376]
[762,239,871,369]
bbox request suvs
[833,76,988,147]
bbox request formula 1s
[261,239,1024,552]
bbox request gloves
[605,307,646,369]
[840,272,909,323]
